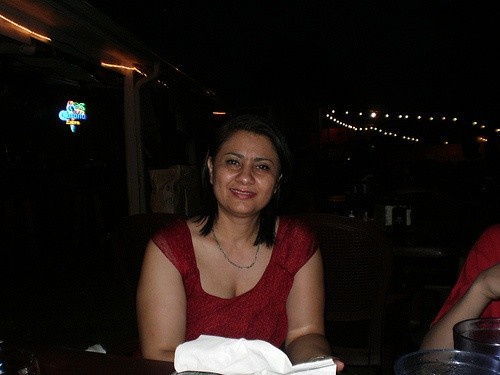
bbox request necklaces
[212,226,259,268]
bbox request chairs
[287,214,394,365]
[110,213,192,296]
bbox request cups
[0,347,41,375]
[452,318,500,351]
[394,348,500,375]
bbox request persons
[418,223,500,370]
[136,114,345,374]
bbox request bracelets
[314,356,326,359]
